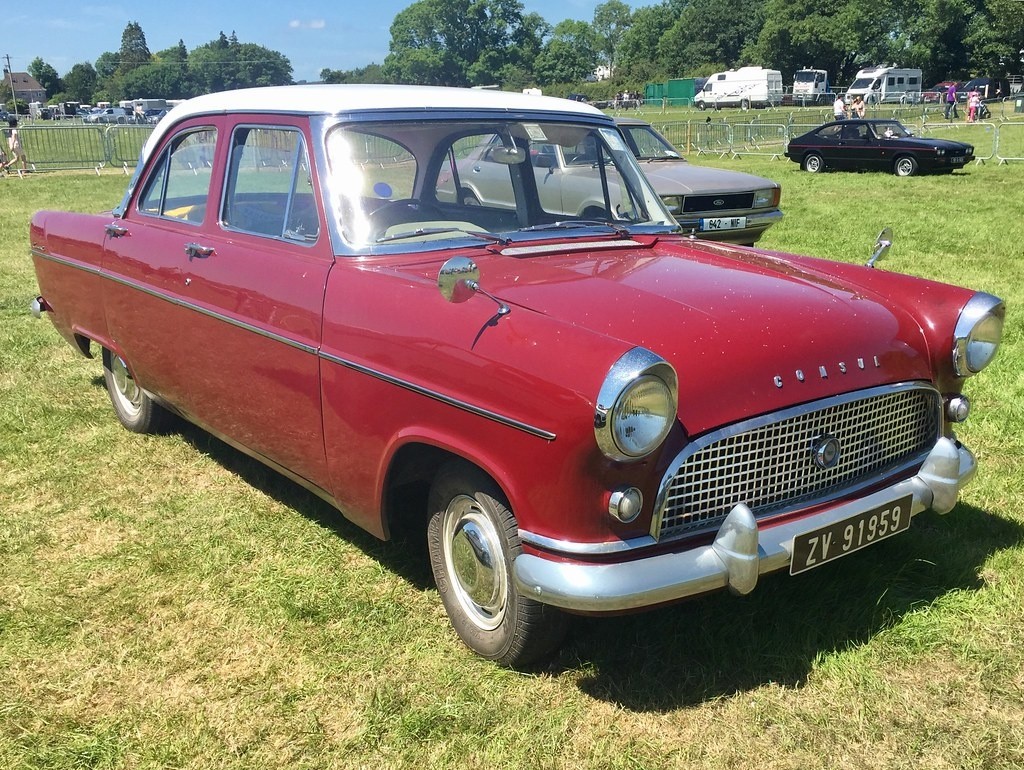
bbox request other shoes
[21,171,29,174]
[3,165,10,174]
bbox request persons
[967,85,981,122]
[135,103,143,121]
[834,94,846,121]
[852,96,866,119]
[615,90,638,111]
[945,80,959,119]
[0,118,33,176]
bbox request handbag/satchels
[2,129,12,139]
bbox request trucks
[565,93,608,110]
[0,103,22,122]
[792,69,847,107]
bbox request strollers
[975,98,992,119]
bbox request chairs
[844,124,859,139]
[536,145,562,168]
[867,123,877,139]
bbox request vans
[955,77,1011,103]
[844,64,923,104]
[693,66,783,111]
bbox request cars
[920,81,965,103]
[436,115,784,248]
[28,83,1009,668]
[784,118,975,177]
[36,101,168,124]
